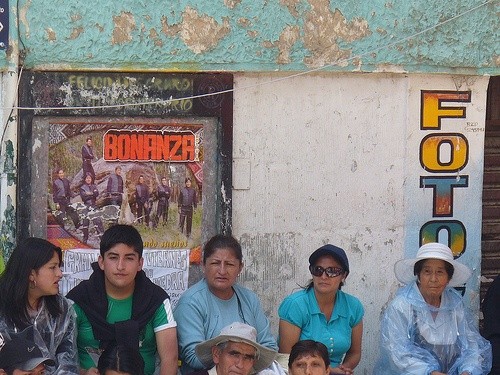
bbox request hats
[309,244,349,273]
[0,340,55,371]
[195,323,278,374]
[394,242,470,287]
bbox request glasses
[309,264,345,278]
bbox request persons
[0,237,79,375]
[374,242,493,375]
[0,339,56,375]
[97,343,145,375]
[155,177,171,228]
[288,340,330,375]
[195,321,278,375]
[53,137,124,245]
[178,178,197,239]
[171,234,279,375]
[483,273,500,375]
[278,244,364,375]
[55,224,178,375]
[135,175,151,231]
